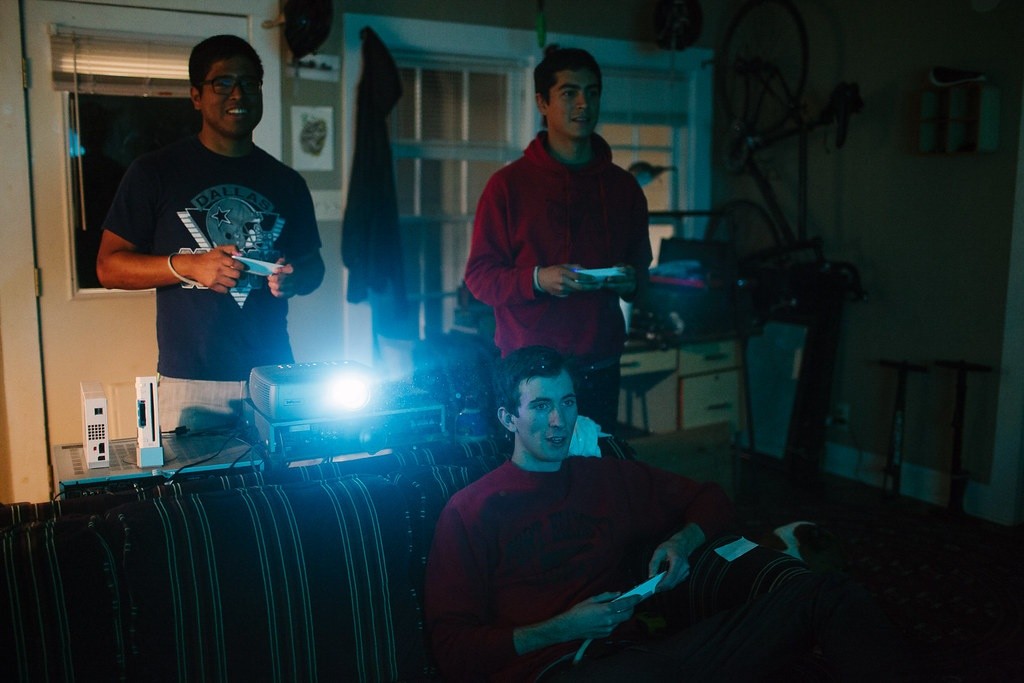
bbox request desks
[59,445,486,500]
[451,324,765,444]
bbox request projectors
[249,359,380,417]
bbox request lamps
[628,161,689,239]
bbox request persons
[96,35,327,436]
[426,344,930,683]
[464,47,654,432]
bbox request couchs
[1,442,846,683]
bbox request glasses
[201,77,263,96]
